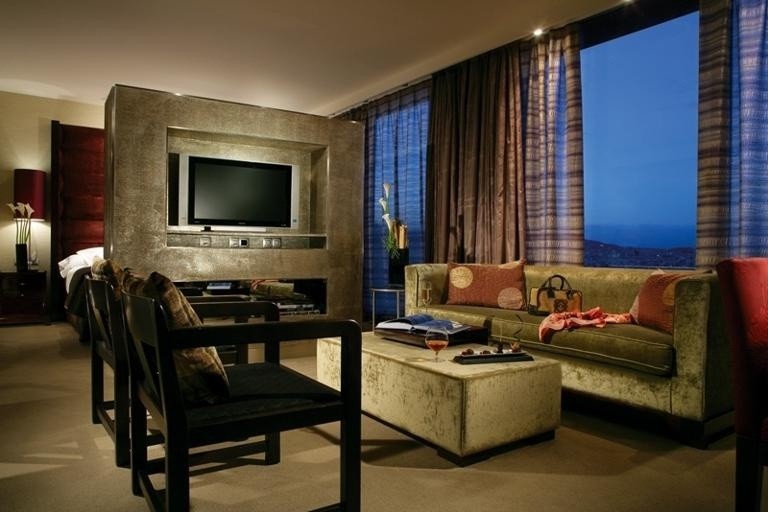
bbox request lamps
[13,168,46,222]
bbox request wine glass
[421,280,432,313]
[424,325,449,364]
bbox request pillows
[58,246,104,278]
[443,256,528,312]
[89,256,231,405]
[628,267,713,335]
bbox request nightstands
[0,270,51,325]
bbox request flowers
[377,182,401,259]
[6,202,35,245]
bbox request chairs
[714,255,768,512]
[83,281,357,510]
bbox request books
[373,313,472,335]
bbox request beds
[63,268,108,344]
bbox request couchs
[403,262,737,450]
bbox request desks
[369,287,405,331]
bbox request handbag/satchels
[525,273,584,316]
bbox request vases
[389,248,409,285]
[16,244,28,272]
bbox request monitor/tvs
[178,152,299,232]
